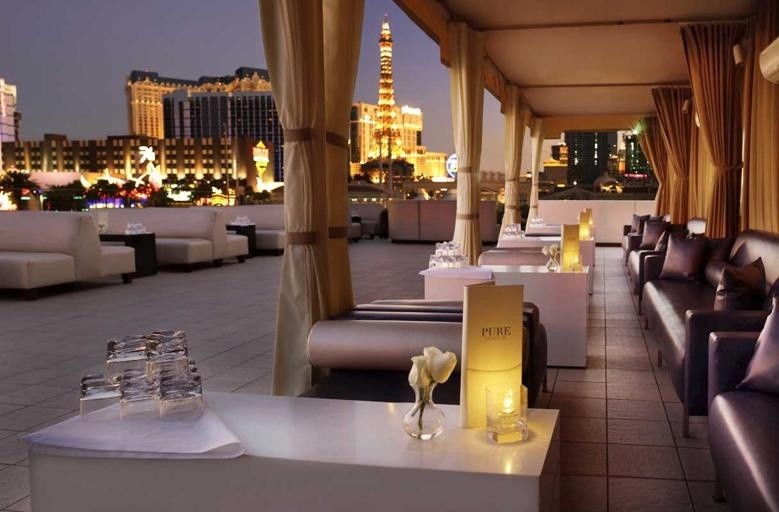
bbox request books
[460,282,524,430]
[560,224,579,269]
[579,207,592,239]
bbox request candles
[495,406,520,429]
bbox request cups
[485,383,528,445]
[503,223,525,240]
[569,254,582,272]
[80,330,204,421]
[429,242,468,269]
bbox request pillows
[659,232,708,282]
[631,214,651,232]
[654,229,689,251]
[713,256,766,310]
[637,218,662,234]
[640,221,671,250]
[734,295,779,393]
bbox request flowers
[407,346,457,430]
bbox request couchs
[0,211,136,301]
[388,200,500,241]
[622,214,671,267]
[348,204,384,239]
[91,208,249,271]
[707,331,779,511]
[347,212,361,242]
[190,204,284,257]
[642,231,779,438]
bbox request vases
[401,384,446,441]
